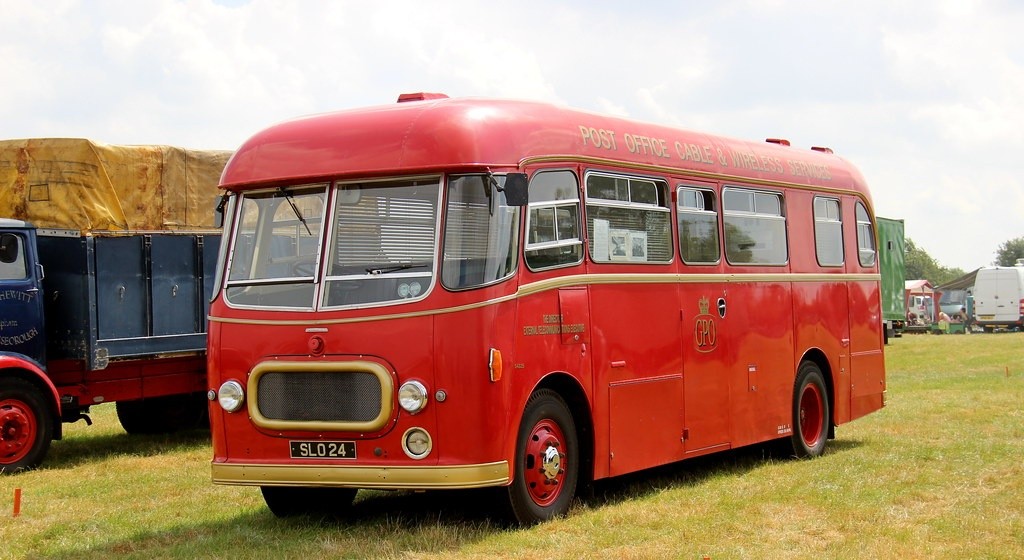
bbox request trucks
[0,218,318,475]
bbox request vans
[975,266,1024,333]
[909,295,932,318]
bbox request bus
[206,93,887,527]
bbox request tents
[905,279,941,326]
